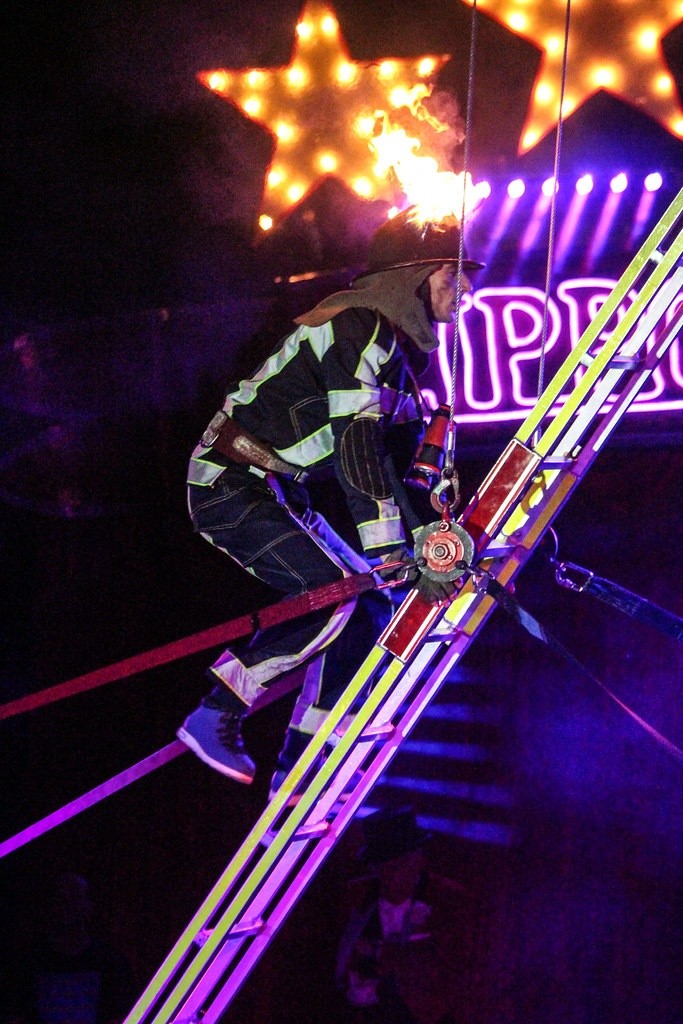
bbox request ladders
[122,190,683,1024]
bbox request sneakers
[268,767,372,806]
[176,694,255,785]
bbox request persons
[176,206,486,808]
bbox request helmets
[368,204,486,271]
